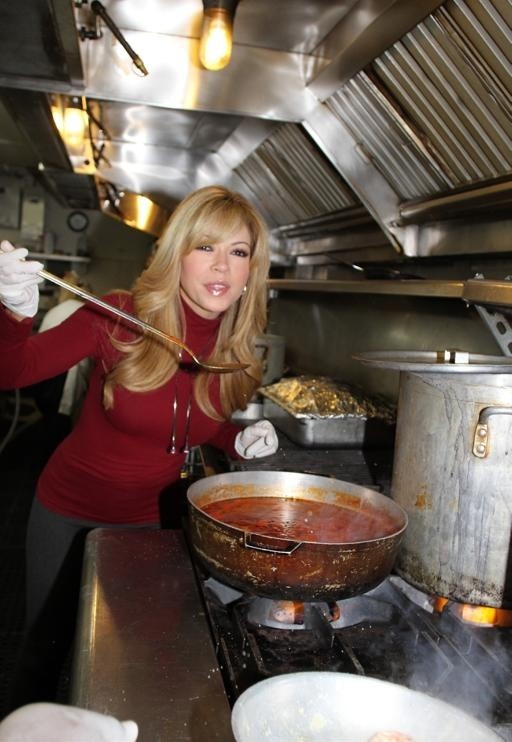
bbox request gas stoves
[195,567,512,742]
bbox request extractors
[214,1,511,269]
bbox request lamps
[199,1,237,73]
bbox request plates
[230,672,505,742]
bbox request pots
[390,364,512,611]
[185,470,409,605]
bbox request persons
[1,183,275,715]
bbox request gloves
[1,247,43,318]
[235,420,278,459]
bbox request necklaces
[170,324,219,455]
